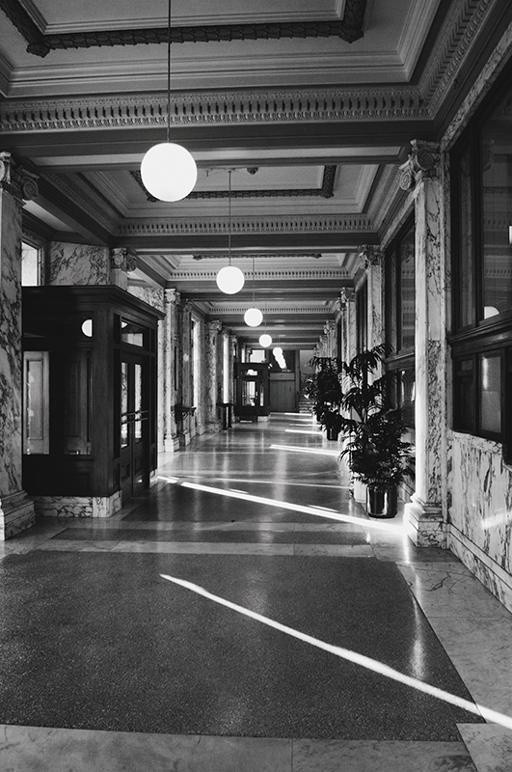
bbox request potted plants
[315,342,417,520]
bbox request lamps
[243,256,264,327]
[215,170,245,297]
[251,331,293,372]
[141,0,199,200]
